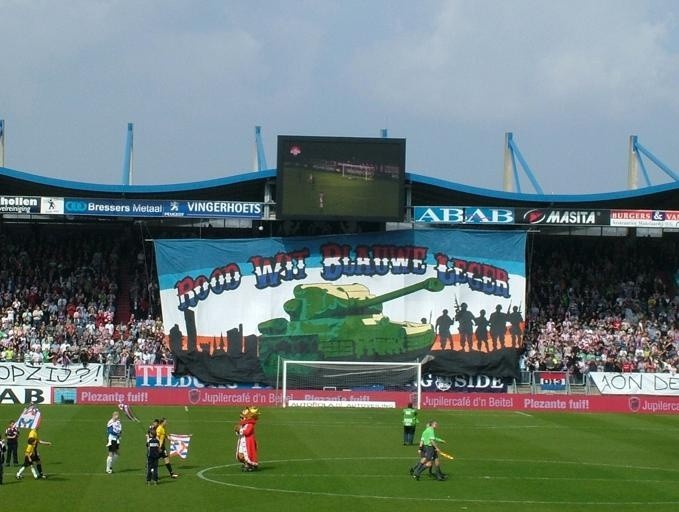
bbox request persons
[409,420,449,481]
[106,411,122,474]
[400,402,419,446]
[0,436,7,485]
[145,429,160,487]
[421,302,523,352]
[4,420,20,467]
[0,227,174,365]
[319,191,325,213]
[29,429,51,479]
[308,172,315,190]
[519,239,679,384]
[147,419,160,432]
[151,417,178,479]
[16,438,41,480]
[106,415,122,447]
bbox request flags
[118,402,137,422]
[168,434,191,459]
[12,405,43,430]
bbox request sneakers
[4,462,48,480]
[408,468,449,481]
